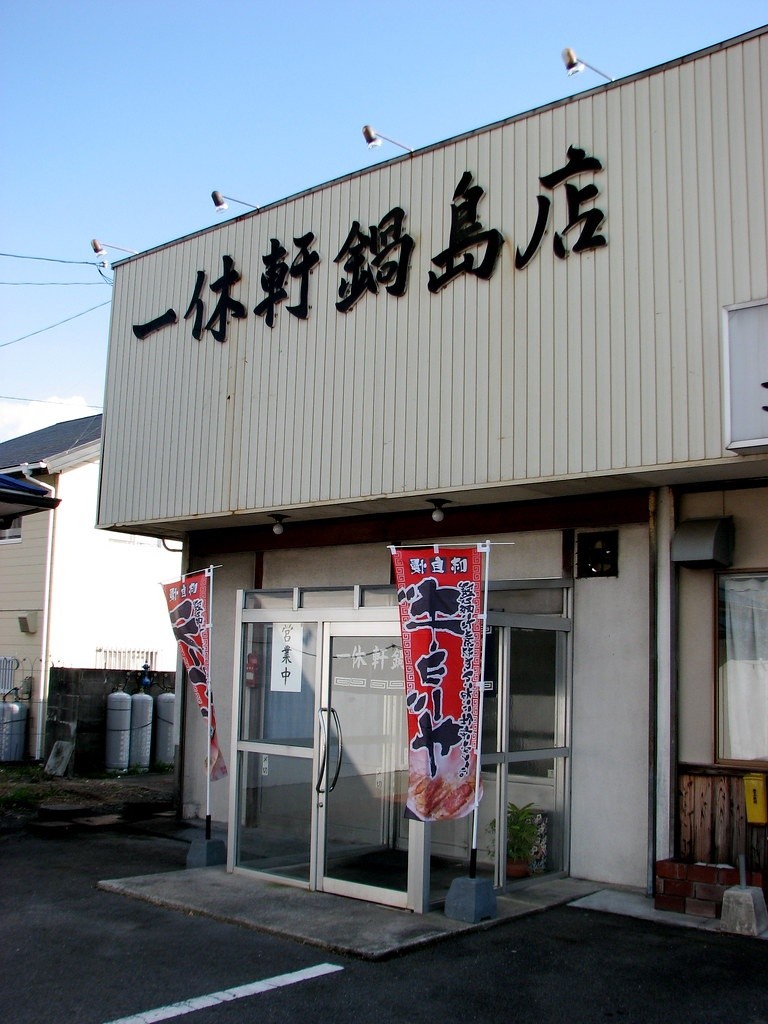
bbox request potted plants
[486,801,538,880]
